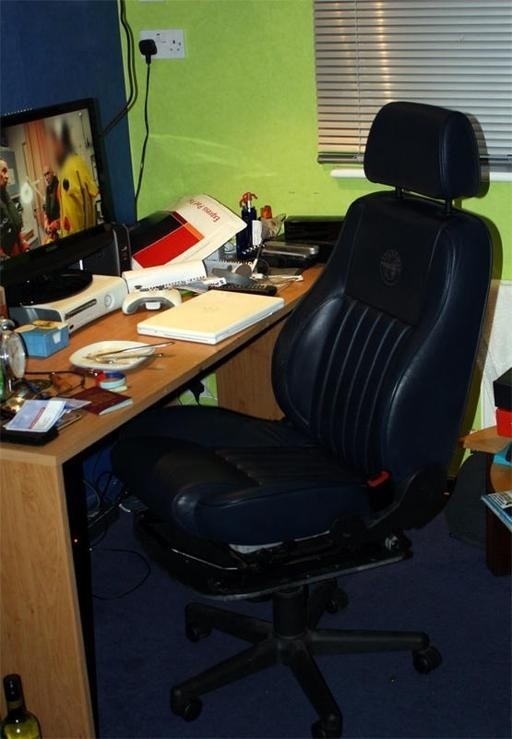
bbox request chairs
[110,103,493,739]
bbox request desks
[1,265,327,739]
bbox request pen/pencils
[248,238,265,271]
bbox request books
[70,386,133,416]
[122,259,249,293]
[481,489,512,534]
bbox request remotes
[210,282,277,297]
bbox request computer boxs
[6,273,129,334]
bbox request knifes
[87,341,175,360]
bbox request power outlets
[140,29,185,59]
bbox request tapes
[96,373,126,389]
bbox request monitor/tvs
[1,98,117,306]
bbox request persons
[0,119,99,263]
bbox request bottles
[0,674,43,738]
[237,192,277,260]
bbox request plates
[71,339,154,371]
